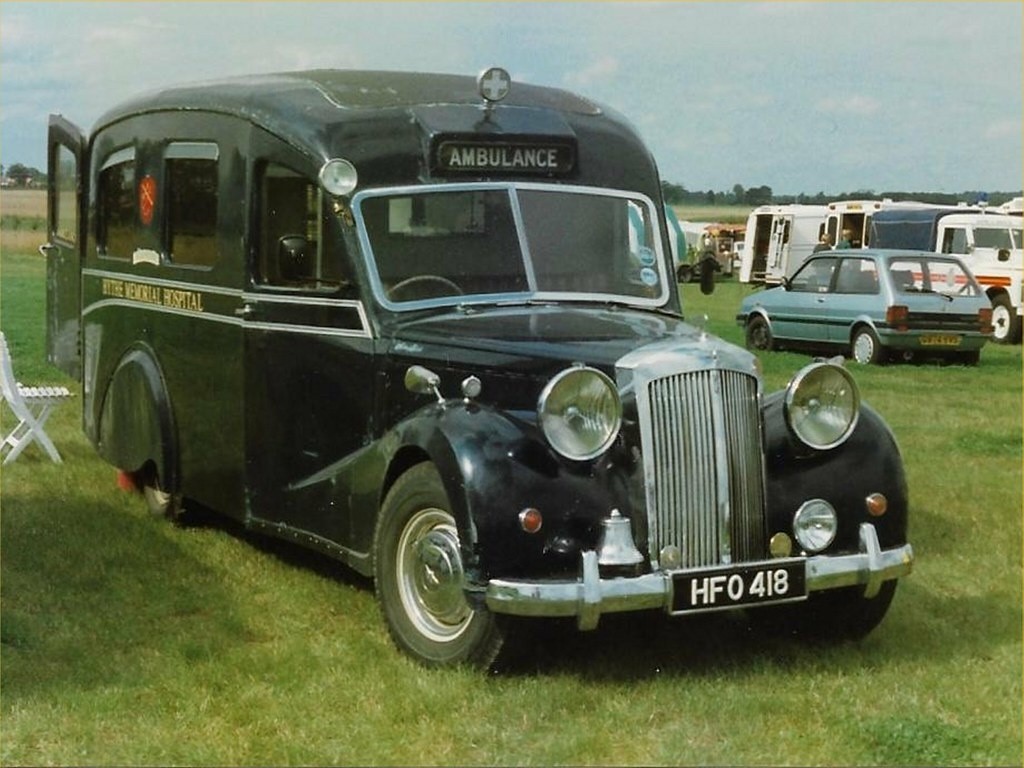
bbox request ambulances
[43,68,918,685]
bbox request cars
[735,249,995,366]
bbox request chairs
[850,270,876,293]
[892,270,919,293]
[0,332,78,467]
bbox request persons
[834,229,853,271]
[813,234,833,291]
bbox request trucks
[868,207,1024,345]
[823,200,967,249]
[739,203,828,288]
